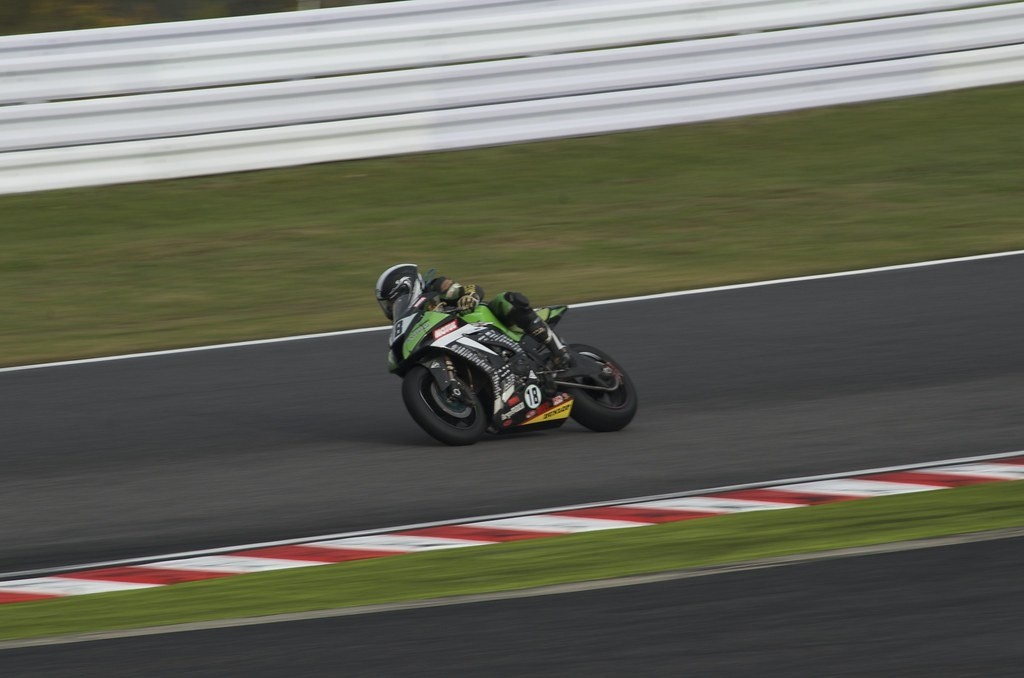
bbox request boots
[527,318,570,370]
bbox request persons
[375,264,571,369]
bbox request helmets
[376,262,425,323]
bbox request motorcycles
[388,269,639,446]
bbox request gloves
[456,291,479,316]
